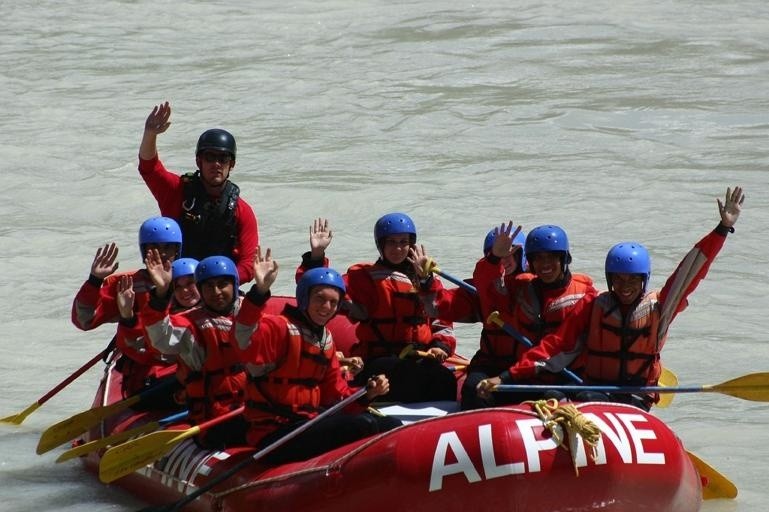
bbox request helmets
[525,224,571,275]
[195,256,240,311]
[484,226,527,274]
[196,129,236,155]
[375,213,417,262]
[140,216,183,261]
[172,257,199,277]
[296,268,348,312]
[606,242,651,293]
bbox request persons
[72,216,183,412]
[141,248,249,449]
[406,225,538,412]
[475,186,745,412]
[138,101,260,284]
[115,257,206,416]
[295,212,458,402]
[229,244,390,469]
[473,220,594,384]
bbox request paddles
[486,309,738,502]
[99,357,359,484]
[35,394,139,456]
[481,372,768,403]
[5,341,117,424]
[141,375,380,511]
[427,258,679,407]
[57,411,188,466]
